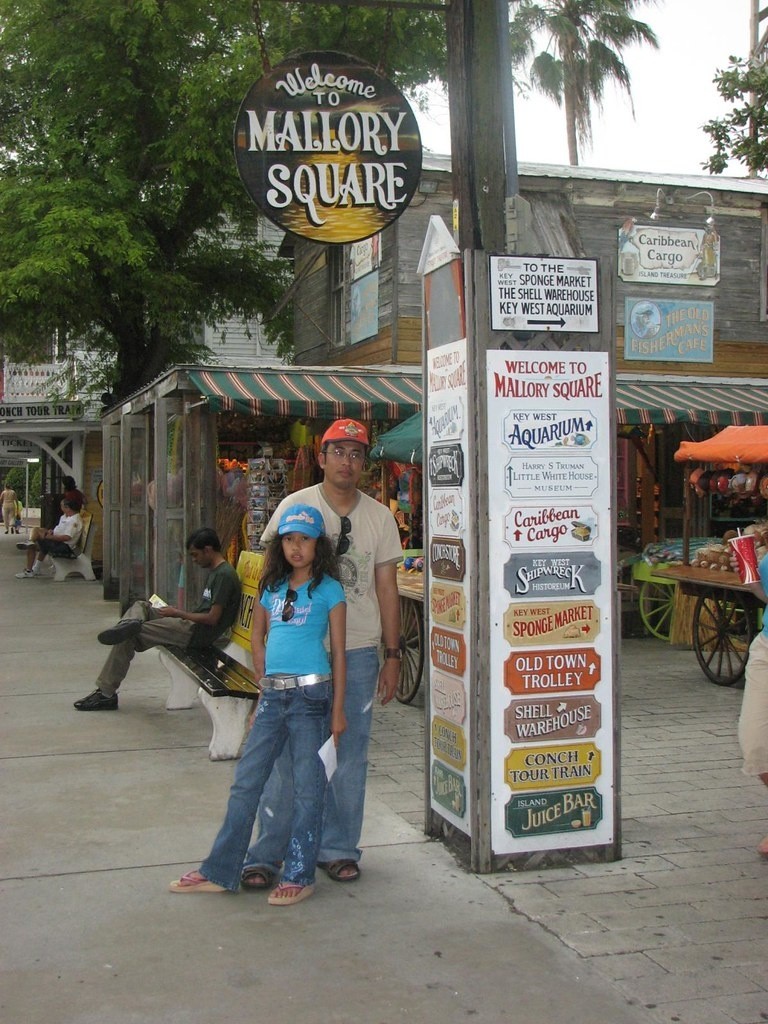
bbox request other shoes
[11,528,14,534]
[15,530,20,534]
[32,567,41,574]
[5,531,8,534]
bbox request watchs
[384,648,403,663]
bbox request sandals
[317,858,360,880]
[241,866,274,887]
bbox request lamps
[684,190,716,226]
[417,178,439,194]
[650,188,674,221]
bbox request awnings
[181,366,768,427]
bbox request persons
[239,419,405,887]
[729,547,768,855]
[0,483,18,534]
[62,476,86,511]
[73,528,240,711]
[14,498,84,579]
[168,504,347,904]
[13,497,23,534]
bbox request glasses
[282,589,297,621]
[338,517,351,554]
[321,450,367,462]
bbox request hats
[322,419,368,445]
[277,504,326,539]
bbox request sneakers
[16,541,36,550]
[15,570,34,578]
[74,687,118,710]
[97,619,142,645]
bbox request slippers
[169,871,227,893]
[268,883,314,905]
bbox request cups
[727,535,761,585]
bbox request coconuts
[691,529,768,573]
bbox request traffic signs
[489,254,599,334]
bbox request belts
[258,674,332,691]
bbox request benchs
[155,550,271,760]
[48,510,97,582]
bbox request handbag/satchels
[15,519,21,527]
